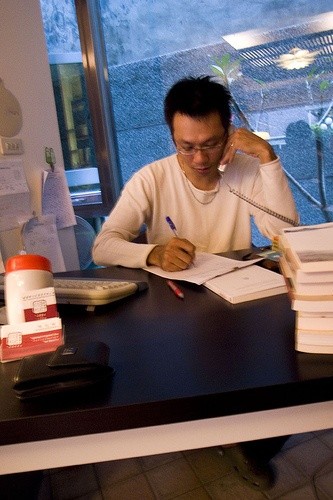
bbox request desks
[0,246,333,477]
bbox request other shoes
[224,445,278,490]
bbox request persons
[92,74,298,272]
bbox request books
[278,222,333,354]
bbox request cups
[4,255,53,323]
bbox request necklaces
[178,156,221,205]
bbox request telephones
[217,124,235,175]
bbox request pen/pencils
[165,215,196,267]
[167,280,185,299]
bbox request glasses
[173,133,229,155]
